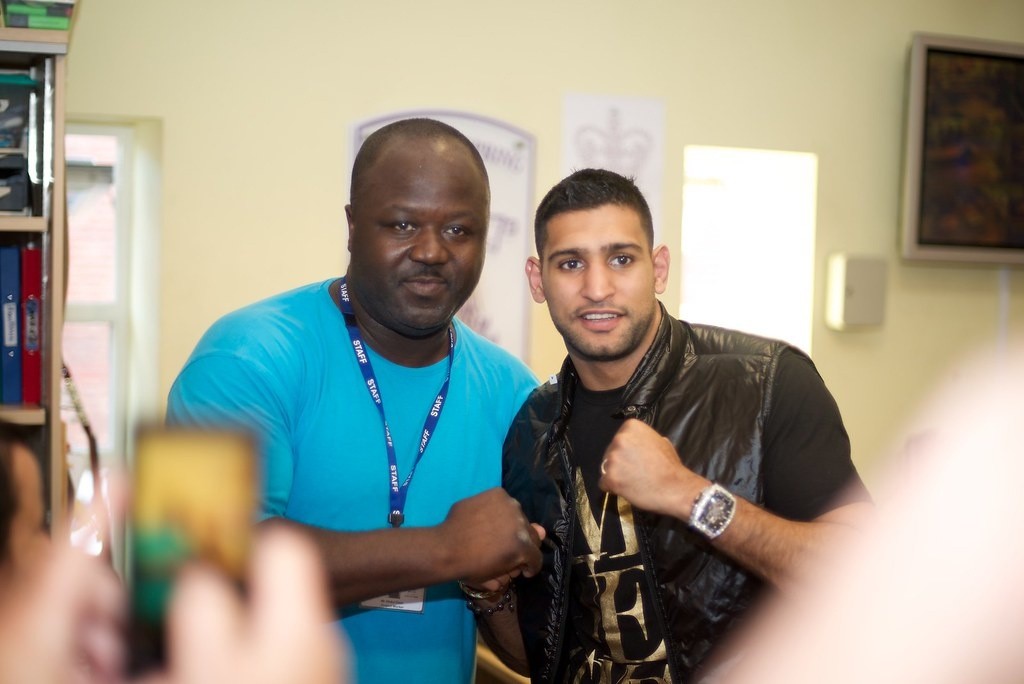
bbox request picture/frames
[900,32,1023,264]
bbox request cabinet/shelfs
[0,0,72,543]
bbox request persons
[0,346,352,684]
[165,117,544,684]
[696,326,1024,683]
[457,169,869,684]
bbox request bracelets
[457,578,505,599]
[466,588,514,616]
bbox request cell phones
[128,423,261,675]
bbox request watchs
[688,482,737,541]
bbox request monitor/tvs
[900,33,1024,269]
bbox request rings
[600,458,607,476]
